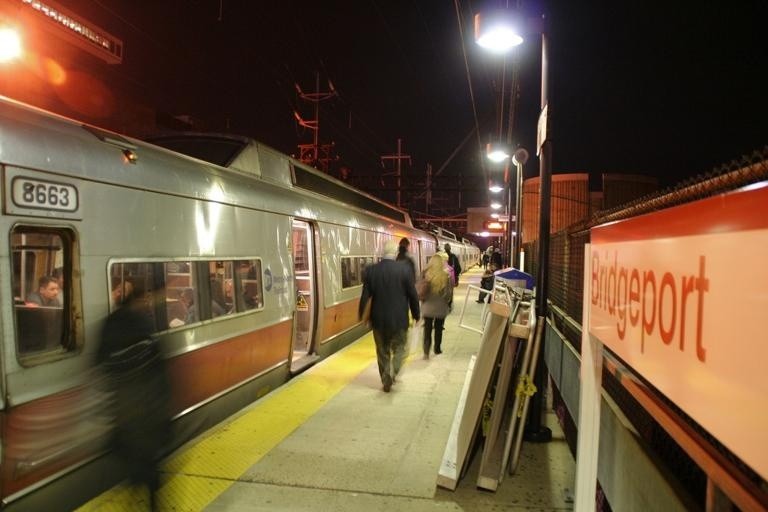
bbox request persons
[475,264,496,304]
[357,239,423,393]
[29,266,260,358]
[96,284,177,511]
[417,253,453,360]
[480,248,502,271]
[394,237,462,330]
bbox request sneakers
[381,349,443,394]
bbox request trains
[0,92,481,511]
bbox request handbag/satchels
[415,276,432,301]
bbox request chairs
[15,272,259,352]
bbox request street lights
[477,7,561,443]
